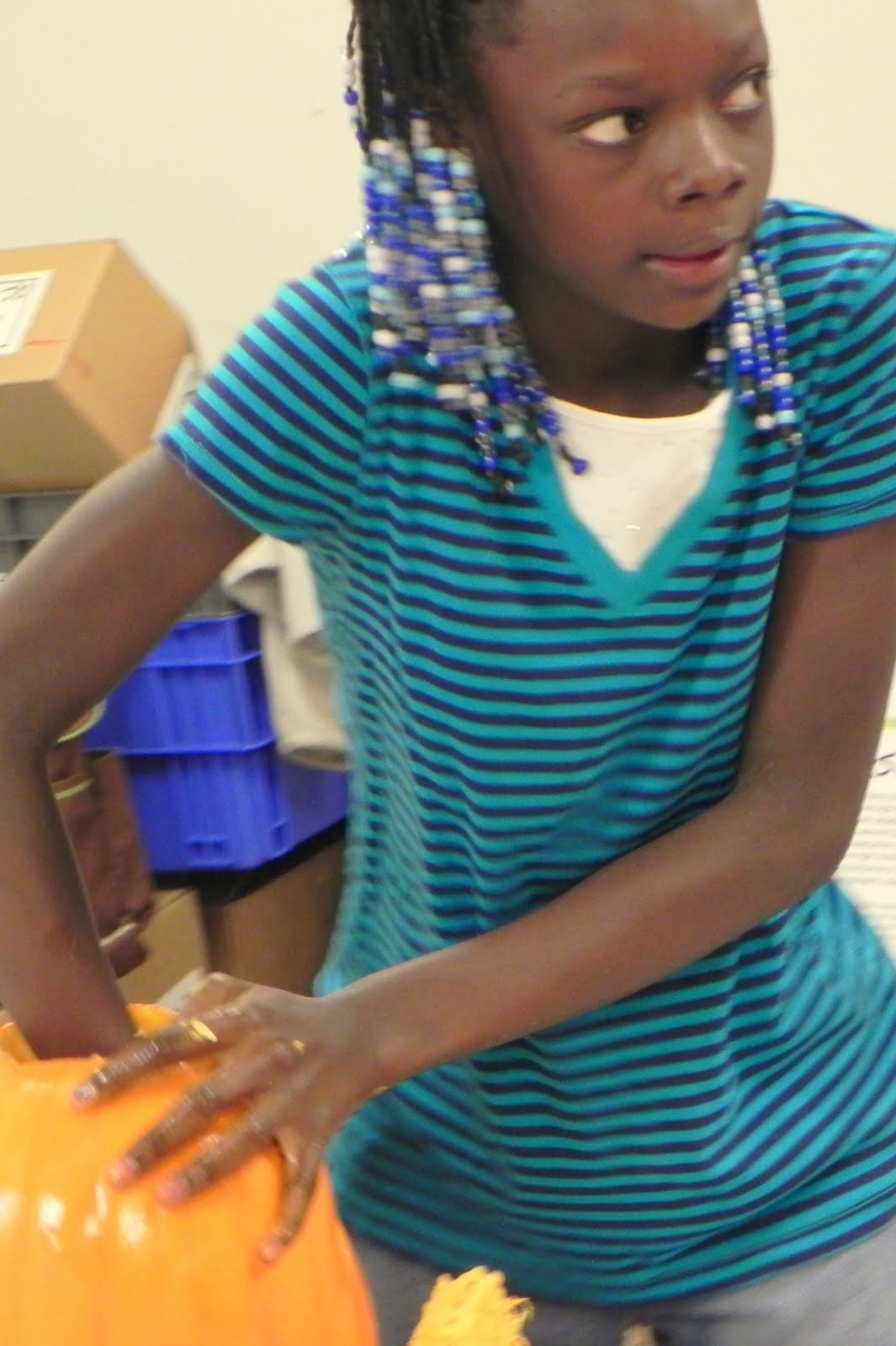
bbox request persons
[0,0,896,1346]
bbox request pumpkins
[0,1003,379,1346]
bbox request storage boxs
[101,885,217,1008]
[202,842,346,1000]
[77,613,276,754]
[128,750,349,877]
[0,236,200,495]
[0,489,245,616]
